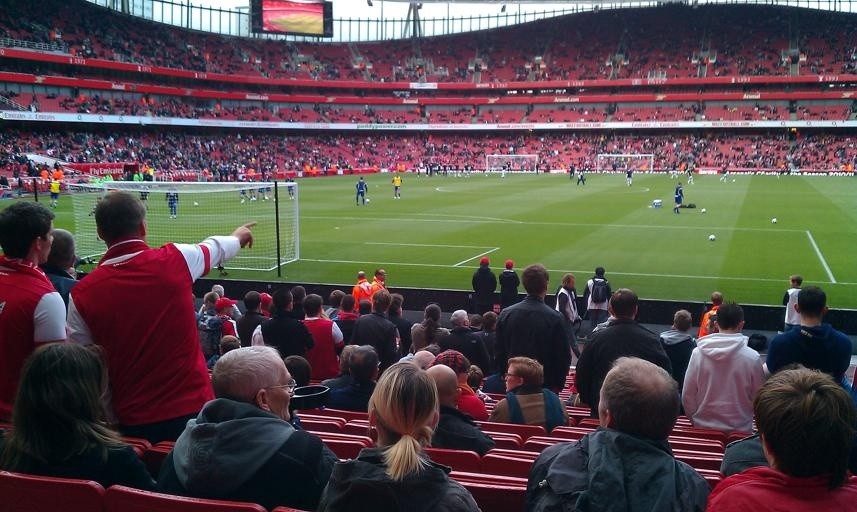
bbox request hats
[260,293,272,305]
[427,348,465,375]
[506,260,513,268]
[214,297,237,310]
[480,257,490,265]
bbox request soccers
[701,208,706,213]
[366,198,370,204]
[194,201,199,206]
[709,234,716,240]
[771,217,777,224]
[732,179,736,183]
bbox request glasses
[268,379,297,393]
[504,372,521,381]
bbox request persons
[0,256,857,512]
[0,1,857,217]
[38,228,81,324]
[64,189,259,444]
[0,200,69,431]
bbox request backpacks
[591,278,610,303]
